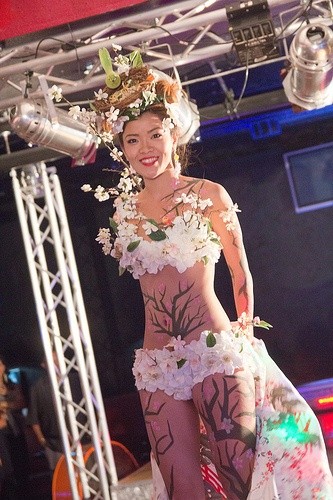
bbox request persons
[96,66,333,500]
[0,351,75,500]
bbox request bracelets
[37,437,45,443]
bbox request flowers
[47,44,241,401]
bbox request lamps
[10,70,98,169]
[282,23,333,114]
